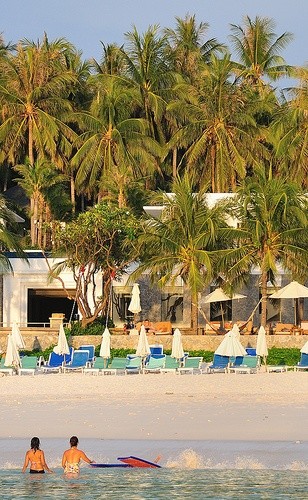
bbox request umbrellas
[257,325,269,373]
[170,328,184,375]
[197,287,247,329]
[3,334,22,376]
[215,323,249,369]
[268,280,308,327]
[299,341,308,354]
[57,321,70,374]
[136,324,152,373]
[11,320,26,350]
[99,326,112,369]
[128,282,142,328]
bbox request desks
[290,329,303,336]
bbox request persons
[61,436,96,480]
[21,436,52,475]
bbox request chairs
[108,320,308,335]
[0,345,308,376]
